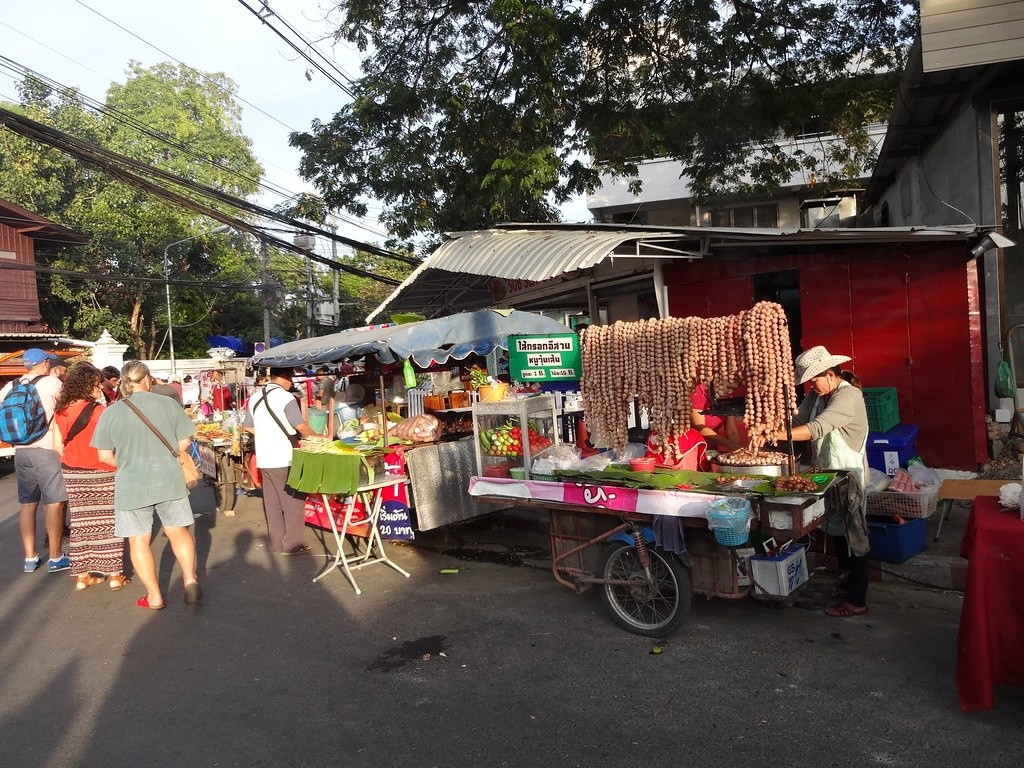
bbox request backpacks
[0,375,55,445]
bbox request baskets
[529,471,560,482]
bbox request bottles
[403,357,416,388]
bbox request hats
[51,354,72,368]
[23,349,57,369]
[792,345,852,385]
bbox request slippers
[826,603,868,617]
[822,590,843,599]
[183,584,203,604]
[136,594,165,610]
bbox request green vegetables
[469,368,487,390]
[501,416,541,433]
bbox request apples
[507,428,551,457]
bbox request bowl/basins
[485,466,510,478]
[509,466,525,479]
[629,458,655,473]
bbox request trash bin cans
[709,498,751,546]
[309,410,328,434]
[865,424,920,478]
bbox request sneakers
[48,553,71,572]
[24,552,43,572]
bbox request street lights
[164,224,229,377]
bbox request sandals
[75,572,105,591]
[110,574,131,591]
[282,545,311,555]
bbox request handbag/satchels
[178,451,198,491]
[288,434,298,448]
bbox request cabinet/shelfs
[472,394,559,481]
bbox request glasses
[148,375,153,381]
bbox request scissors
[806,475,828,484]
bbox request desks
[956,495,1024,714]
[405,437,514,542]
[935,479,1024,542]
[312,474,410,595]
[516,409,585,443]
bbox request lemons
[500,430,519,457]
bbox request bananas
[479,430,494,452]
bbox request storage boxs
[860,386,899,434]
[865,519,929,564]
[867,483,938,519]
[765,495,826,529]
[866,425,921,477]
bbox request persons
[0,349,71,572]
[249,367,329,555]
[151,371,183,408]
[574,323,588,332]
[747,346,869,616]
[251,369,269,394]
[45,354,70,547]
[168,375,199,408]
[291,360,365,412]
[209,383,234,410]
[688,378,741,453]
[89,361,203,610]
[102,366,120,407]
[238,382,250,410]
[52,361,131,590]
[498,349,509,374]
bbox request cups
[222,411,229,421]
[209,413,214,421]
[213,408,223,421]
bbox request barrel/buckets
[478,383,509,403]
[375,388,388,402]
[308,406,328,434]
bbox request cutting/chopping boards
[939,479,1022,500]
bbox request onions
[488,445,506,456]
